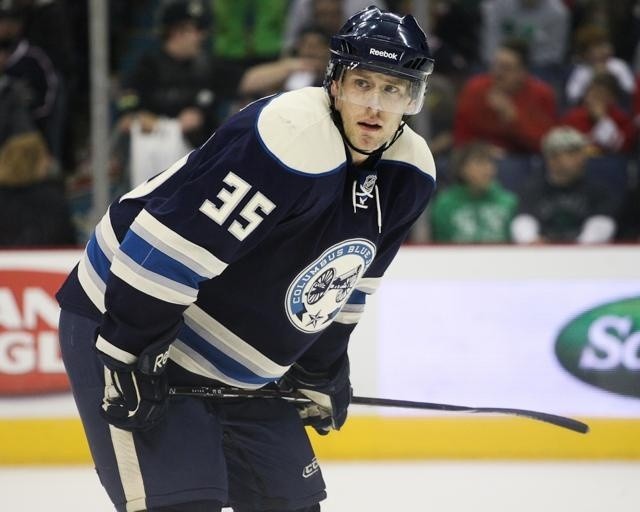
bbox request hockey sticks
[162,379,589,436]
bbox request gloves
[292,380,354,436]
[322,5,435,115]
[98,361,168,431]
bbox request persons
[55,4,441,511]
